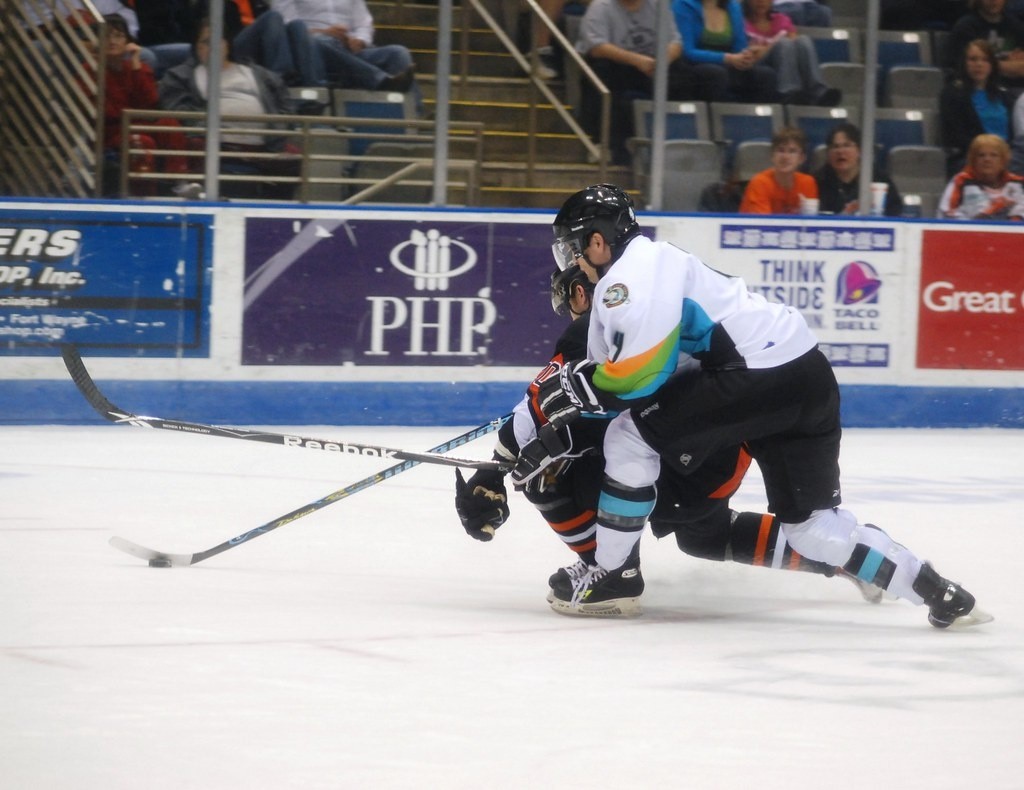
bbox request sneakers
[835,569,899,604]
[547,558,592,603]
[551,539,644,617]
[913,563,994,630]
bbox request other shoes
[588,143,612,165]
[384,61,417,93]
[173,182,201,196]
[819,87,843,106]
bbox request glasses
[199,36,210,45]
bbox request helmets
[552,183,638,260]
[550,263,599,315]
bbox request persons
[455,254,891,602]
[820,123,902,217]
[739,128,819,215]
[698,162,743,213]
[937,134,1024,222]
[519,0,1024,180]
[0,0,424,200]
[548,185,993,632]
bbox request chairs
[504,0,953,218]
[1,0,421,205]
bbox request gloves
[455,466,509,541]
[537,358,609,428]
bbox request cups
[799,197,820,216]
[870,182,889,217]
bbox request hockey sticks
[61,342,515,473]
[108,412,515,570]
